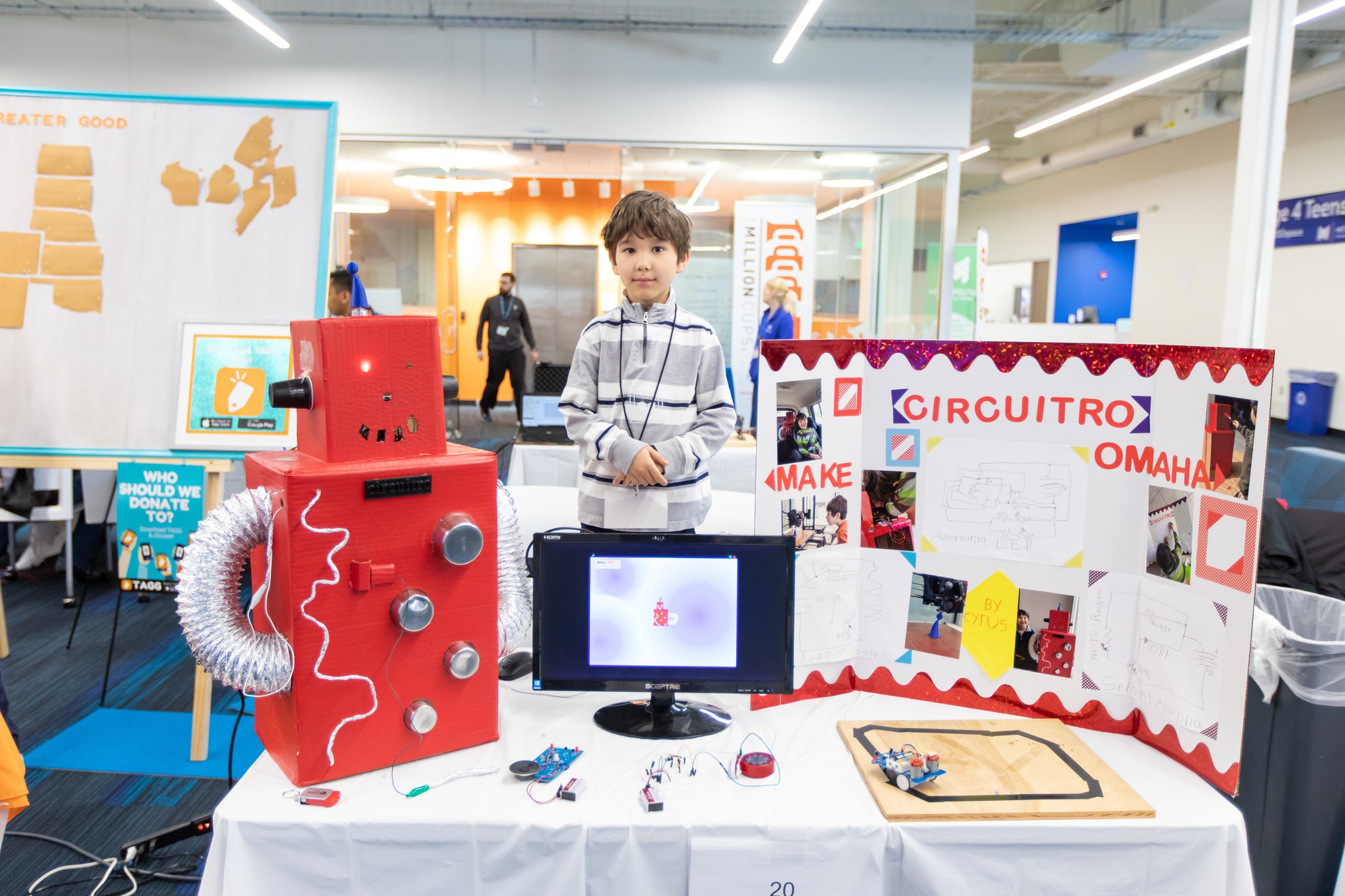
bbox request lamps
[816,1,1345,221]
[213,0,292,50]
[772,0,823,63]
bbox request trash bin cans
[1239,583,1345,896]
[1288,369,1338,433]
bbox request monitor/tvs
[533,532,795,740]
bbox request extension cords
[120,813,213,862]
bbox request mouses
[499,651,533,681]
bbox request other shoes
[475,399,492,422]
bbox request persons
[1233,400,1258,500]
[749,278,796,384]
[558,191,736,533]
[1156,522,1188,584]
[0,512,81,580]
[863,470,916,516]
[826,495,847,545]
[1015,609,1035,670]
[328,269,372,316]
[796,413,822,460]
[476,273,538,425]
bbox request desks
[1277,446,1345,514]
[197,429,1256,896]
